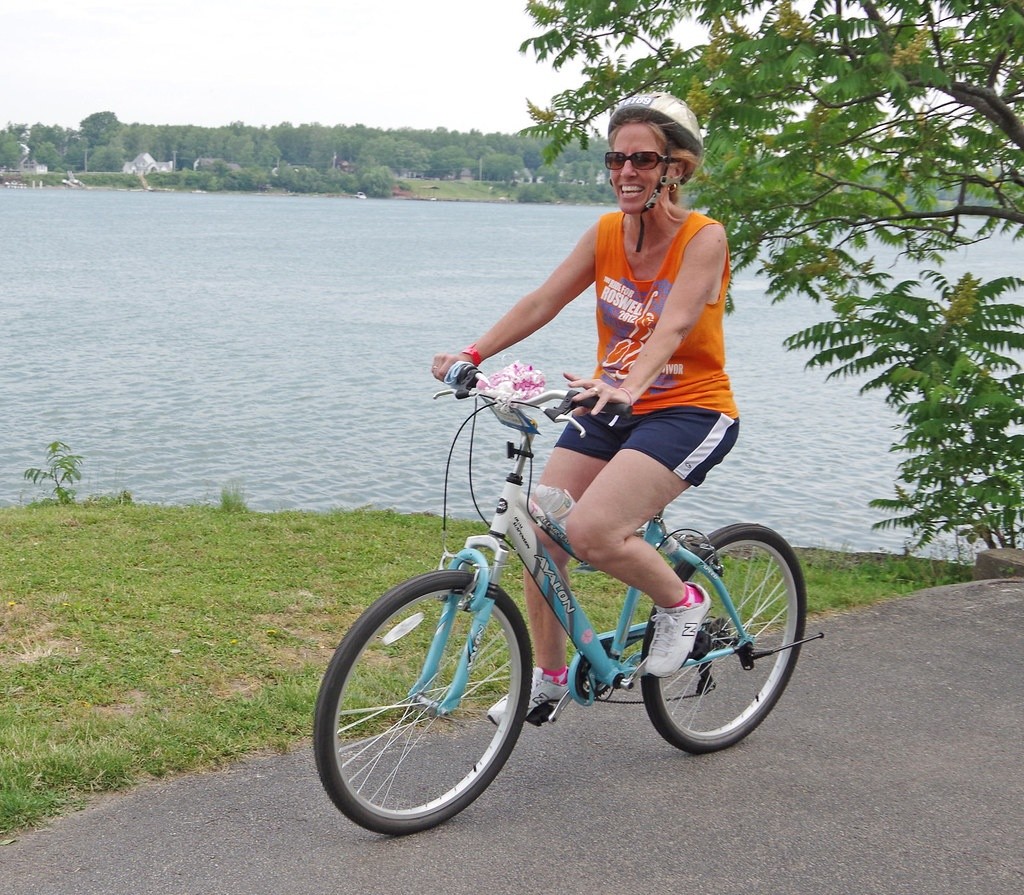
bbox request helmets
[607,94,706,153]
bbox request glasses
[603,146,673,170]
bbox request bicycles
[308,365,809,836]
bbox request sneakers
[641,582,712,676]
[487,667,574,722]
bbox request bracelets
[618,387,633,407]
[461,343,482,367]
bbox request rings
[590,387,598,398]
[432,365,438,369]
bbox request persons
[430,93,740,726]
